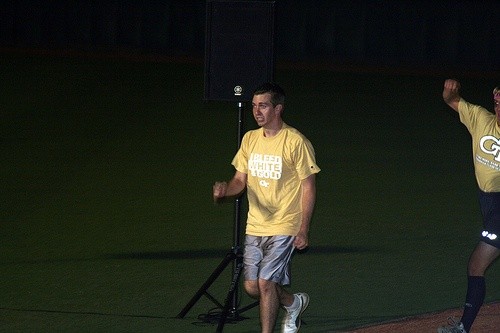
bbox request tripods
[176,102,306,333]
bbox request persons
[439,79,500,333]
[213,84,321,333]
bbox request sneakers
[437,321,466,333]
[281,292,310,333]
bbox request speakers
[203,0,278,103]
